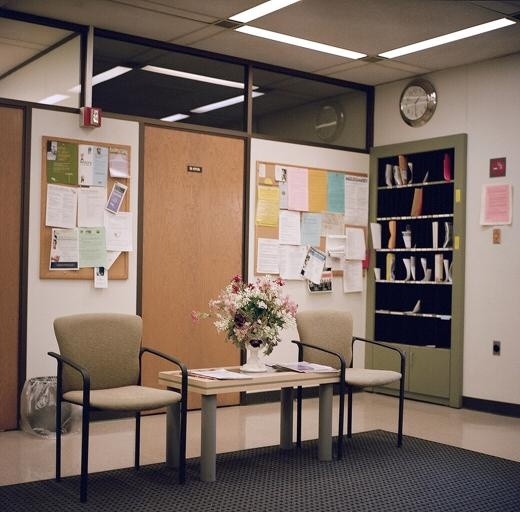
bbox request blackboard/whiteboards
[254,160,369,279]
[39,135,131,282]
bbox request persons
[51,239,60,263]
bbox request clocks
[398,79,438,129]
[312,100,345,143]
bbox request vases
[239,335,268,373]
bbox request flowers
[190,274,299,357]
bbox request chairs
[290,308,407,460]
[47,312,188,503]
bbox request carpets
[0,430,520,512]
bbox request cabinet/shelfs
[361,133,468,410]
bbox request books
[265,360,339,374]
[180,368,254,381]
